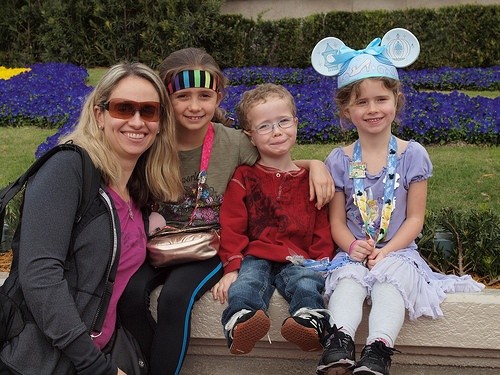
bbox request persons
[0,62,185,375]
[116,48,335,375]
[210,82,335,354]
[286,28,485,375]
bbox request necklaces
[116,184,134,220]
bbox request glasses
[98,97,166,123]
[248,114,296,136]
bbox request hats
[311,27,421,89]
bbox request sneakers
[352,340,402,375]
[281,313,327,352]
[315,323,359,375]
[223,309,270,355]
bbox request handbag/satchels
[146,222,225,267]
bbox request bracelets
[349,240,356,255]
